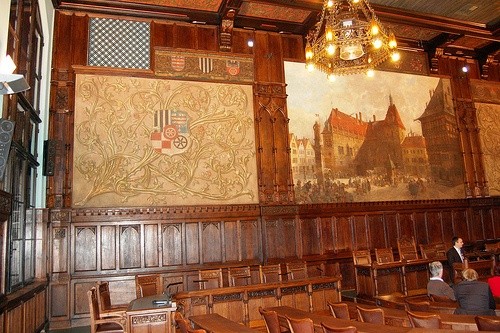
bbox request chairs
[86,237,500,333]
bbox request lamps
[304,0,400,81]
[0,54,31,95]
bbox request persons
[451,262,466,298]
[426,260,455,300]
[447,236,469,287]
[457,268,496,310]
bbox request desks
[354,250,495,306]
[452,260,493,284]
[125,275,343,333]
